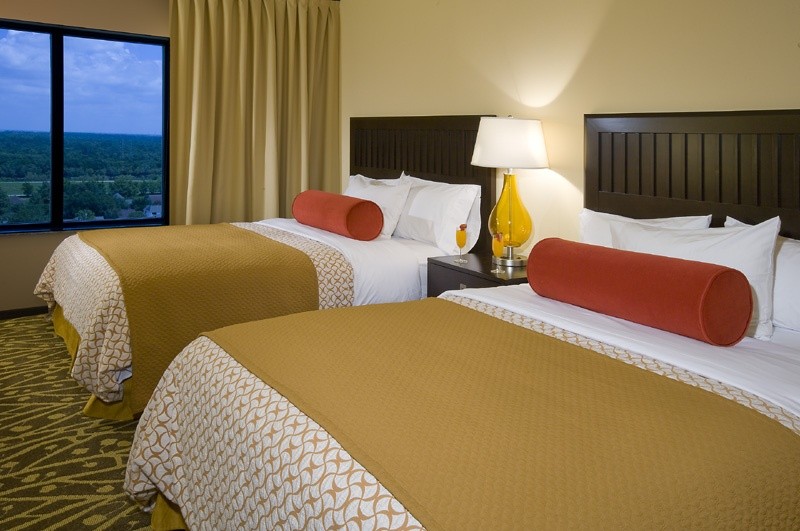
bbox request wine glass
[455,224,467,263]
[492,235,506,273]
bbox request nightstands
[428,253,531,298]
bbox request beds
[123,110,800,531]
[32,115,497,423]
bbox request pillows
[721,216,800,332]
[578,207,711,254]
[345,176,412,239]
[608,215,780,341]
[293,190,384,241]
[395,173,482,256]
[526,237,752,347]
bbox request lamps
[471,117,549,266]
